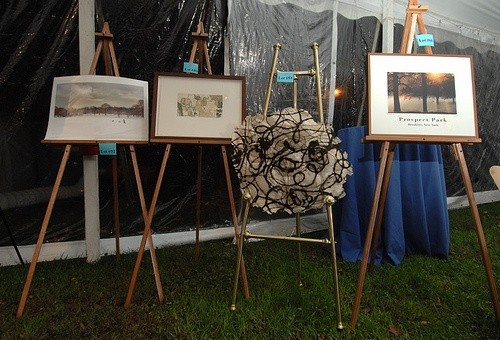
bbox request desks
[322,126,451,265]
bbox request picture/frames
[152,71,246,139]
[368,52,479,139]
